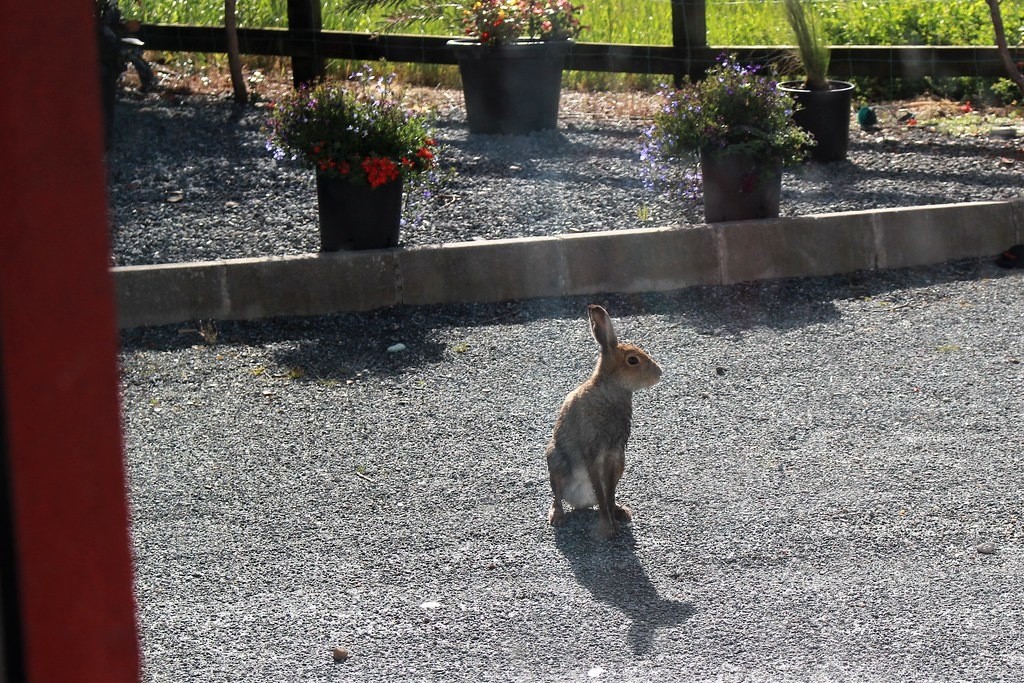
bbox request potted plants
[778,0,856,161]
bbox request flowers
[265,63,462,213]
[370,0,589,44]
[635,51,815,181]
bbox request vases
[447,38,574,135]
[313,158,403,253]
[699,138,783,223]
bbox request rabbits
[546,303,663,539]
